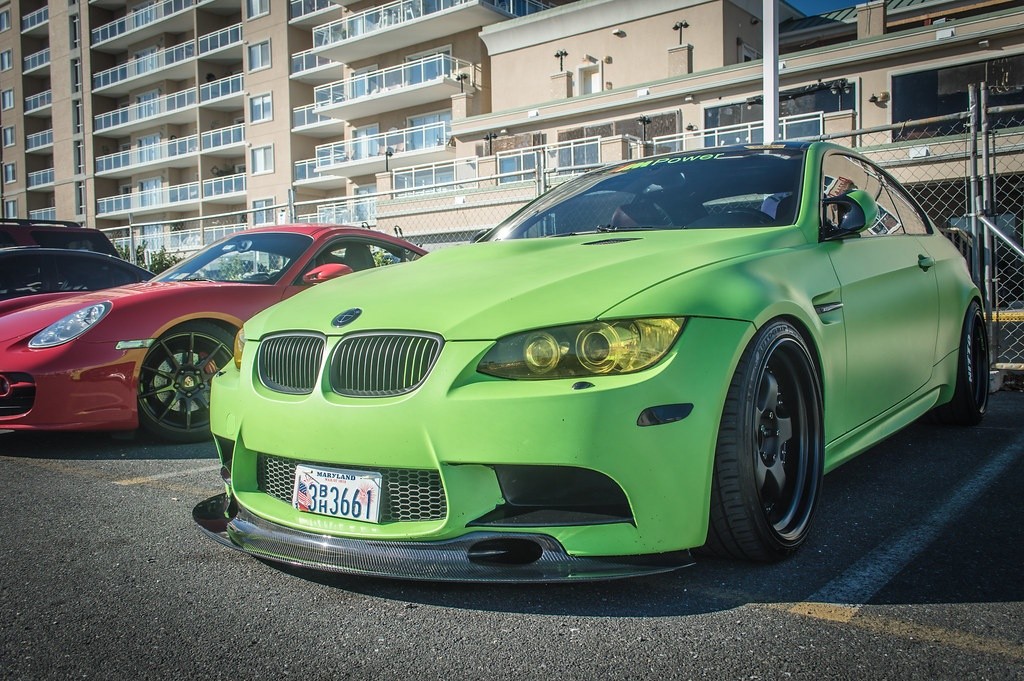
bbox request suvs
[0,219,121,258]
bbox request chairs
[669,200,707,227]
[761,194,798,226]
[611,202,664,225]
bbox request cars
[0,246,157,301]
[192,140,989,582]
[0,222,429,444]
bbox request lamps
[482,132,497,155]
[830,78,851,111]
[636,113,651,141]
[455,71,468,92]
[381,149,393,171]
[554,48,569,71]
[672,18,689,45]
